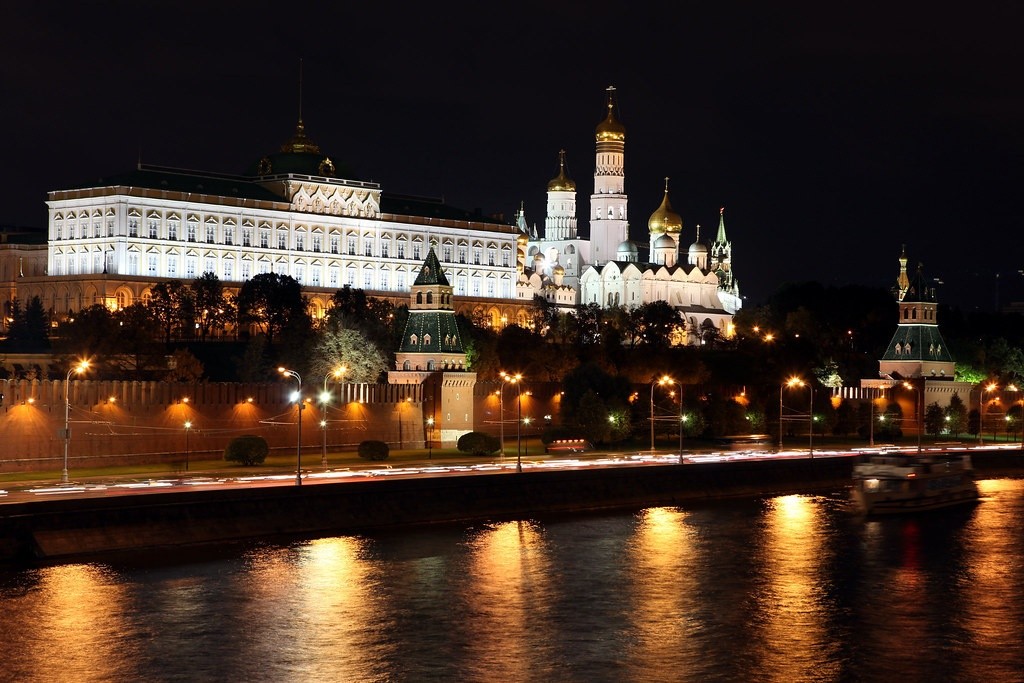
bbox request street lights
[978,382,996,446]
[61,357,88,476]
[276,367,304,481]
[778,377,813,458]
[650,376,684,463]
[499,370,525,470]
[184,422,191,471]
[870,385,883,446]
[319,362,346,461]
[903,381,920,452]
